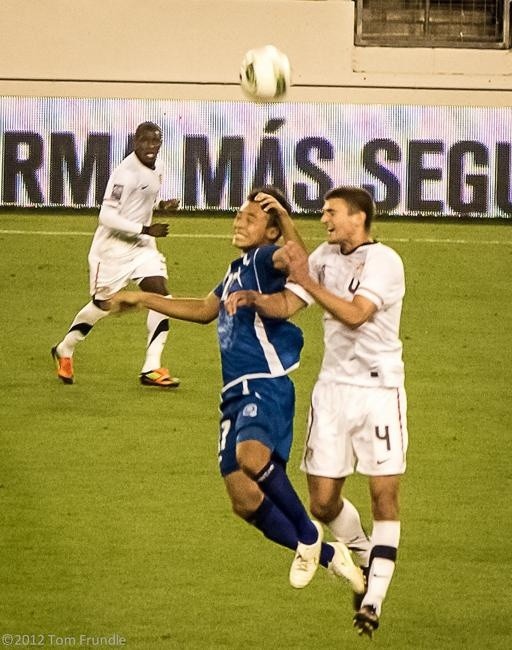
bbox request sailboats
[240,45,291,101]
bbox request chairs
[326,541,366,595]
[354,561,370,612]
[140,367,181,387]
[354,604,379,633]
[50,346,73,383]
[288,518,323,588]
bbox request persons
[224,188,406,637]
[50,122,181,388]
[113,190,368,593]
